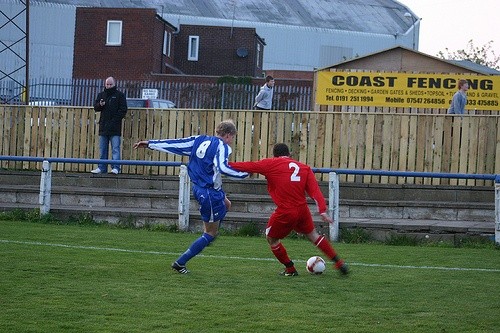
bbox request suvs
[124,98,179,109]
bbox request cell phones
[100,98,104,103]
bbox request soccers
[306,256,326,275]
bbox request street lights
[404,12,415,49]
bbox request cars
[27,101,96,126]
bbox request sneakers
[91,168,102,174]
[340,263,353,274]
[111,168,118,174]
[171,261,190,274]
[278,271,298,277]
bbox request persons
[133,121,249,274]
[432,80,469,150]
[253,76,275,144]
[228,143,349,277]
[90,77,128,175]
